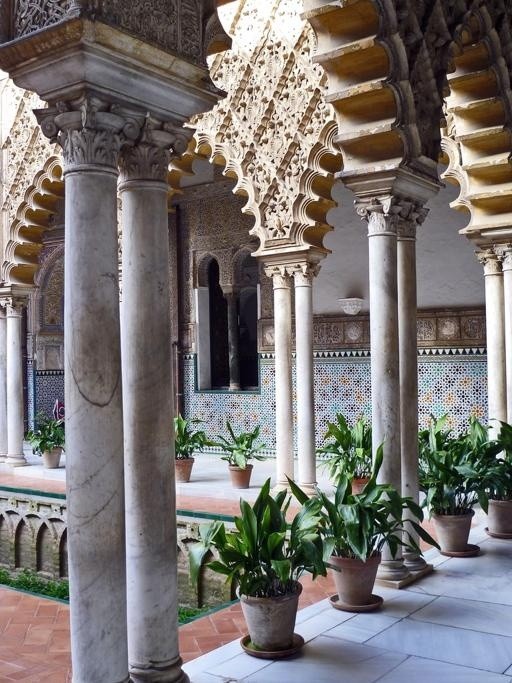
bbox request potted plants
[406,403,487,552]
[209,420,270,491]
[311,409,374,497]
[169,408,216,484]
[185,470,344,652]
[278,435,442,608]
[461,404,512,536]
[21,403,70,473]
